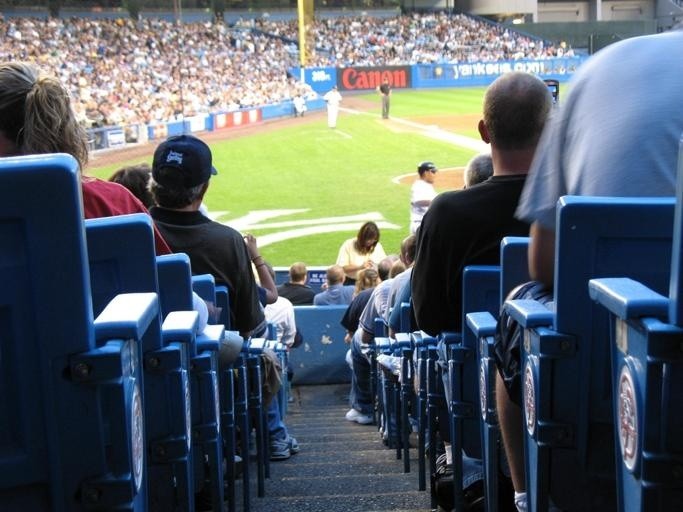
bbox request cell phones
[543,79,558,108]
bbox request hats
[152,135,217,186]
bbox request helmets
[418,162,438,174]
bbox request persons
[337,152,494,465]
[1,0,584,141]
[489,29,682,512]
[110,135,300,461]
[409,70,555,512]
[277,261,357,307]
[0,62,209,335]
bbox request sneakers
[346,408,373,424]
[270,432,299,459]
[452,482,484,512]
